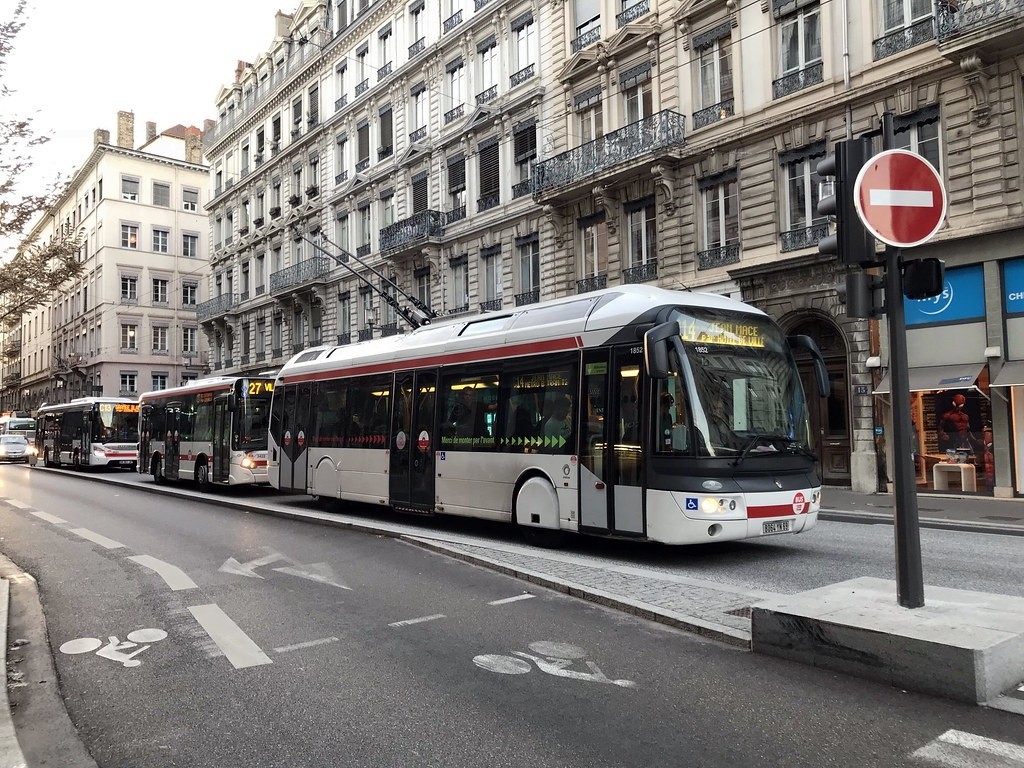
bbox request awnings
[989,361,1024,387]
[872,363,985,396]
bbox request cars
[0,435,34,464]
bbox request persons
[984,432,995,491]
[448,387,497,438]
[701,394,735,454]
[513,392,572,443]
[938,395,983,463]
[661,392,674,451]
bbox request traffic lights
[817,140,875,264]
[903,258,945,301]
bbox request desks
[932,463,977,491]
[922,453,977,482]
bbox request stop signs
[855,149,948,248]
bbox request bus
[137,376,276,491]
[0,417,35,435]
[267,282,833,546]
[34,396,139,470]
